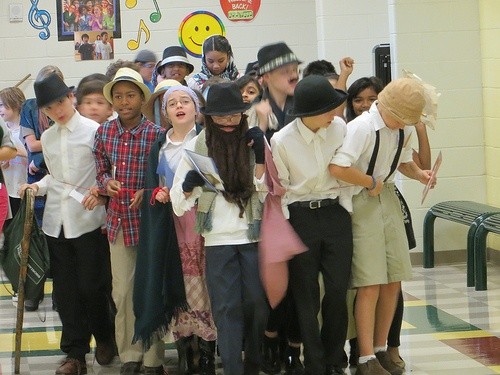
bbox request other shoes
[356,355,391,375]
[290,356,303,369]
[326,363,344,375]
[325,348,349,368]
[51,292,59,312]
[145,363,165,375]
[376,350,403,375]
[24,286,44,311]
[387,347,405,369]
[243,356,260,375]
[55,357,88,375]
[120,361,140,375]
[95,332,116,366]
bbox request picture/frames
[56,0,121,41]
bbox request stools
[423,200,500,291]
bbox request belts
[290,196,340,210]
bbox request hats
[378,77,424,126]
[34,73,75,107]
[101,46,200,116]
[200,83,251,115]
[285,74,349,118]
[246,42,302,74]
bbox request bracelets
[366,175,376,191]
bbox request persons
[0,35,432,375]
[155,85,217,375]
[17,72,116,375]
[95,32,113,60]
[270,75,349,374]
[170,82,271,375]
[328,77,437,375]
[92,66,166,375]
[77,34,96,60]
[62,0,114,31]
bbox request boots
[177,334,196,375]
[348,337,360,365]
[197,334,216,375]
[261,334,282,374]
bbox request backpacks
[0,187,51,297]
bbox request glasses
[214,114,242,124]
